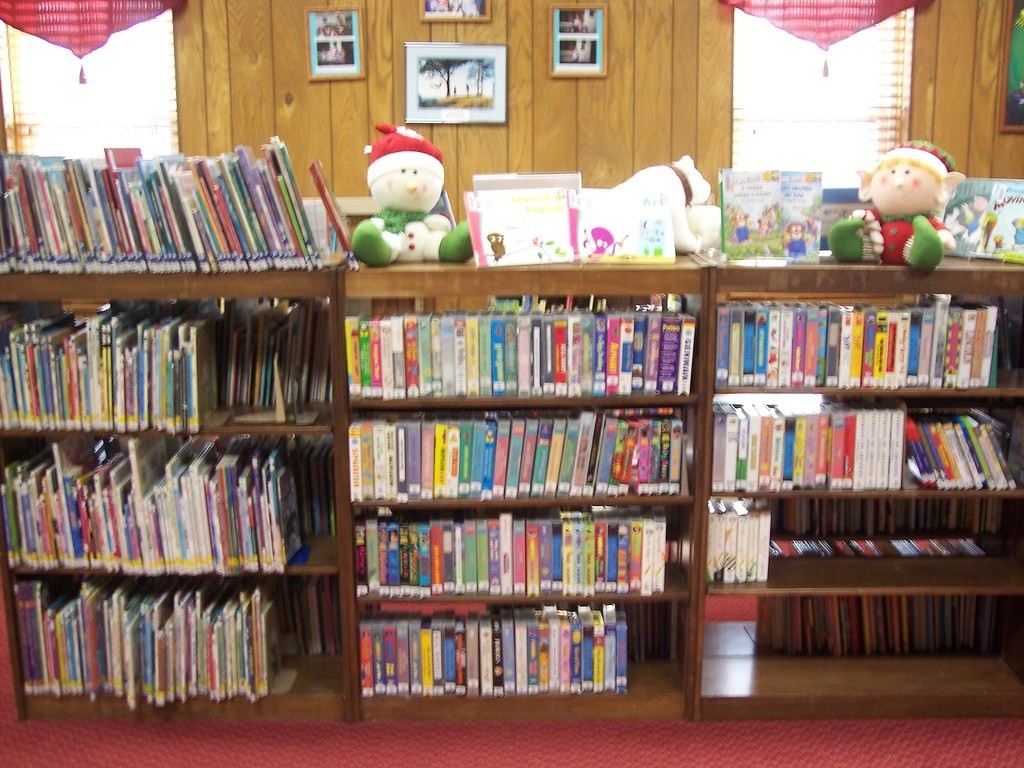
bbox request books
[0,138,1024,709]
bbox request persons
[435,0,453,12]
[320,14,352,65]
[570,14,585,62]
[454,84,469,96]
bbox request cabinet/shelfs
[0,264,354,726]
[697,253,1024,723]
[352,253,712,726]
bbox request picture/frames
[998,0,1024,135]
[403,42,509,127]
[550,3,608,78]
[419,0,492,23]
[304,5,366,82]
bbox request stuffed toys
[828,140,967,273]
[351,121,474,268]
